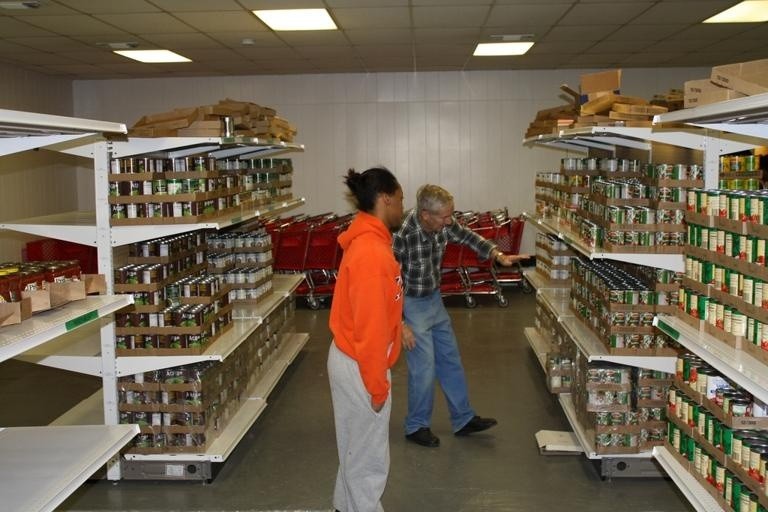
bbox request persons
[327,167,403,512]
[390,184,531,446]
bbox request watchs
[494,253,503,260]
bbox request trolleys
[265,207,527,311]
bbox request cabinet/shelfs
[521,126,758,483]
[1,133,311,486]
[652,93,768,512]
[0,109,141,512]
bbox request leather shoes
[405,428,440,448]
[455,417,498,437]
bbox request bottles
[568,256,681,348]
[130,230,206,257]
[201,296,298,447]
[207,232,275,269]
[0,260,85,324]
[668,348,768,512]
[571,366,673,455]
[719,154,761,192]
[115,262,163,285]
[533,155,700,249]
[533,300,575,392]
[534,232,576,282]
[109,154,294,219]
[162,253,204,277]
[114,304,200,349]
[118,361,202,455]
[163,273,227,299]
[228,266,274,305]
[678,186,768,351]
[220,114,234,138]
